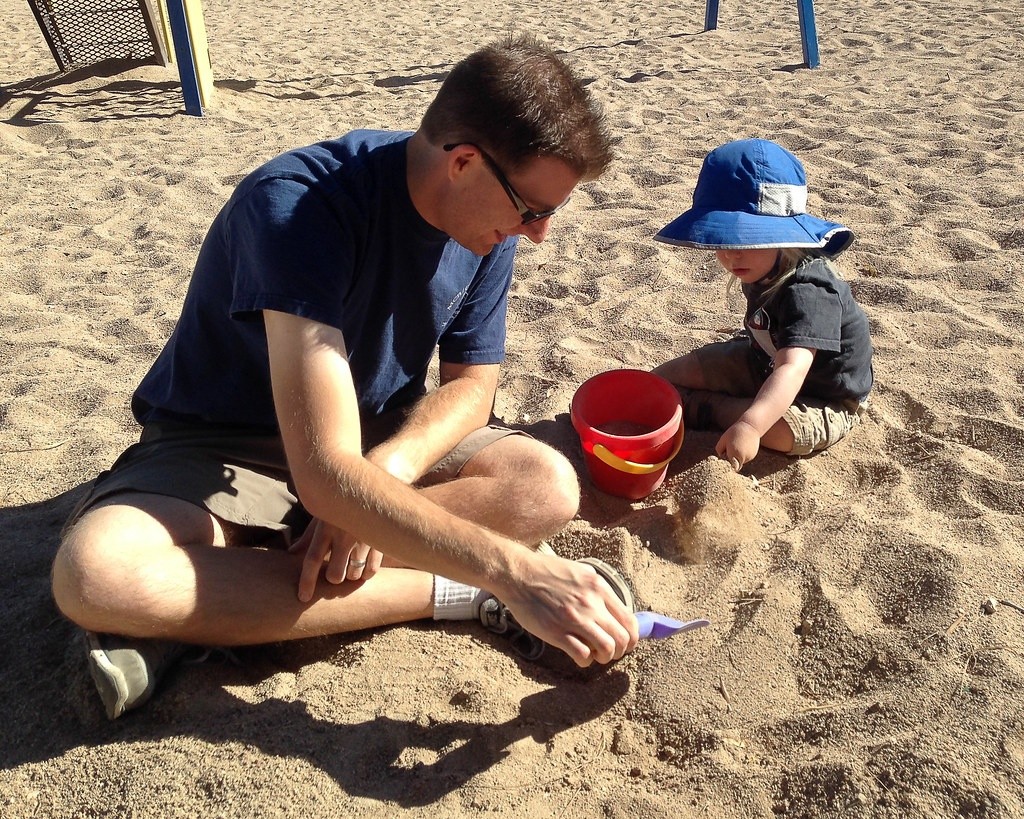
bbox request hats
[652,138,855,263]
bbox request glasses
[443,140,571,227]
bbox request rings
[349,558,366,568]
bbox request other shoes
[86,630,183,722]
[671,383,714,430]
[478,541,637,685]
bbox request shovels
[634,609,709,641]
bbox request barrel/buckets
[571,370,683,499]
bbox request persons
[51,33,639,721]
[649,138,873,474]
